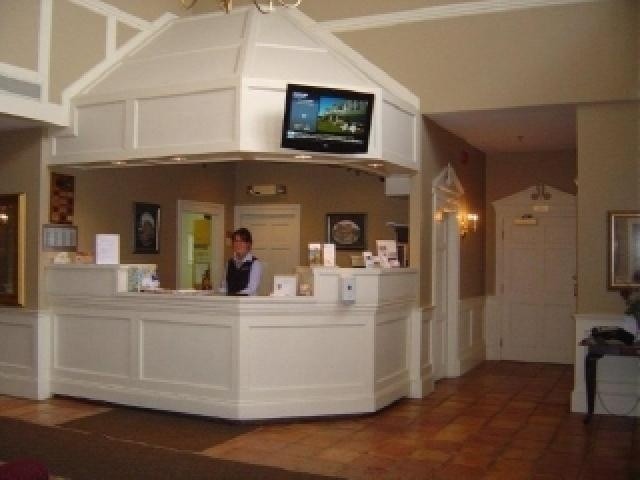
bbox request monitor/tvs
[281,84,375,153]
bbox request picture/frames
[133,202,161,255]
[48,174,75,225]
[607,210,640,291]
[326,211,369,252]
[0,192,26,309]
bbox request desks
[578,341,640,424]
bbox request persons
[214,227,263,297]
[139,219,154,248]
